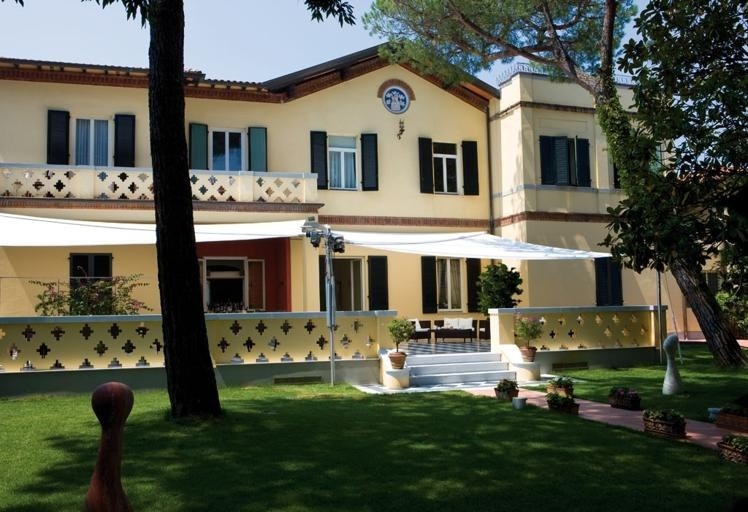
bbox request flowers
[610,387,638,399]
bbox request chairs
[407,320,431,344]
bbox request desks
[435,329,473,344]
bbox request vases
[608,394,641,411]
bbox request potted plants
[643,408,687,439]
[717,432,748,464]
[716,401,748,433]
[545,378,574,397]
[476,262,523,316]
[545,393,579,415]
[494,379,519,401]
[386,316,416,369]
[512,317,545,362]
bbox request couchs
[434,320,490,341]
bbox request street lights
[306,228,345,385]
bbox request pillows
[444,318,458,328]
[458,317,473,329]
[408,319,421,331]
